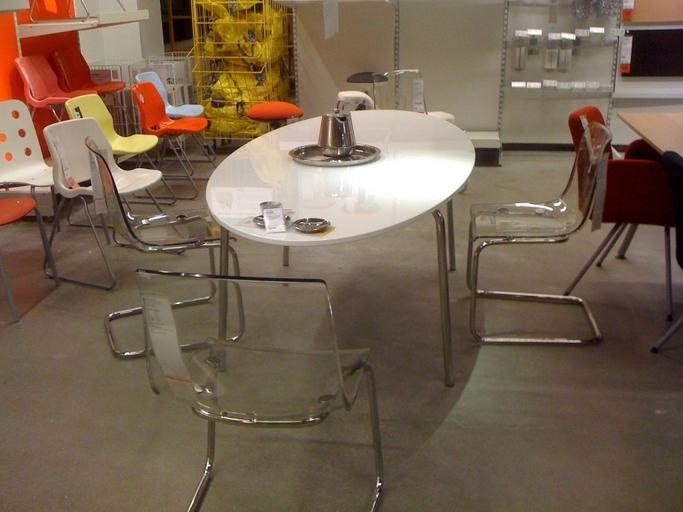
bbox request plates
[254,214,330,233]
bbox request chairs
[42,116,191,292]
[64,91,177,224]
[0,98,63,236]
[50,45,136,137]
[131,268,387,511]
[651,151,683,353]
[372,67,430,115]
[466,121,613,347]
[345,71,388,110]
[71,134,252,361]
[564,106,683,321]
[129,82,219,200]
[3,195,61,323]
[135,70,216,164]
[15,54,98,124]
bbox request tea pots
[318,109,357,157]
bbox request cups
[260,201,286,233]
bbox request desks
[617,111,683,156]
[205,109,477,386]
